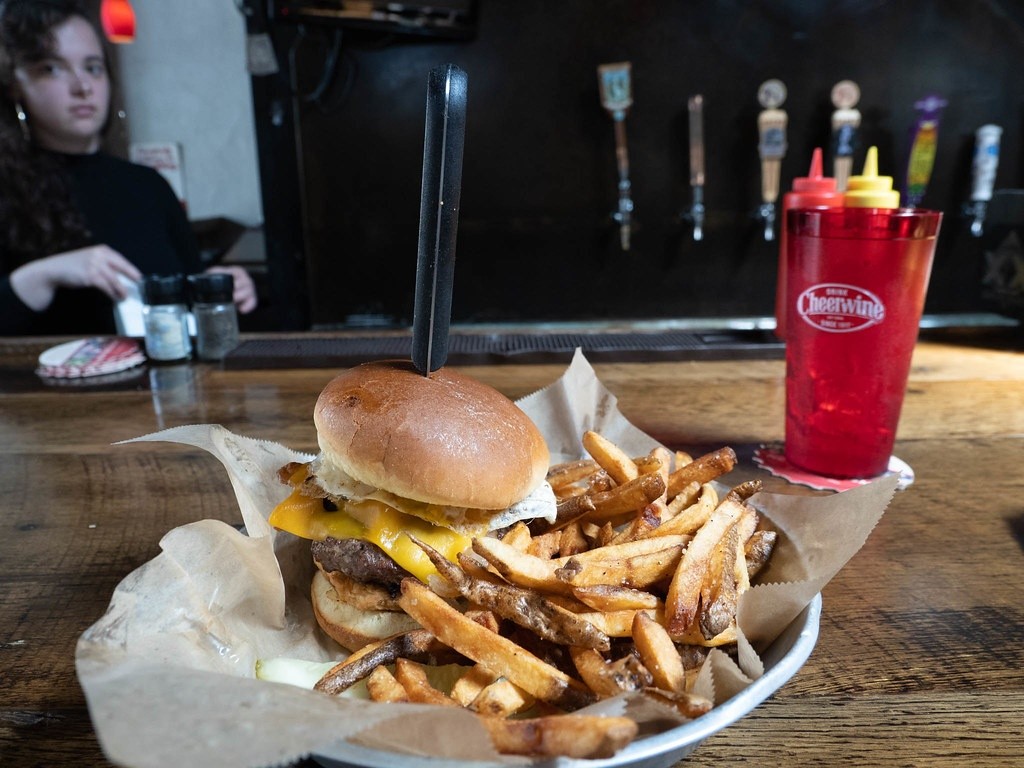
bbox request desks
[0,336,1024,768]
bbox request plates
[182,481,823,768]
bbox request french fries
[255,431,777,761]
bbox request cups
[784,206,944,479]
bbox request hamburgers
[269,359,556,655]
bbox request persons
[0,0,258,336]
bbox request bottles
[775,148,842,343]
[112,273,147,338]
[189,274,243,361]
[140,272,194,360]
[842,146,900,208]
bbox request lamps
[99,1,136,45]
